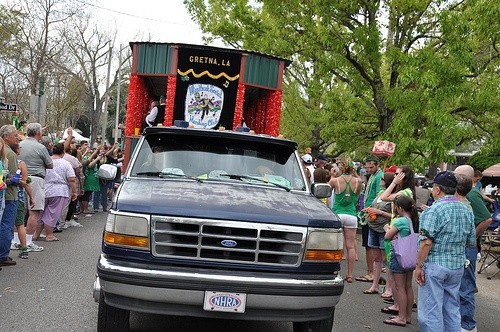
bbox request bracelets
[392,182,397,185]
[415,262,424,268]
[382,211,384,216]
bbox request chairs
[477,226,500,280]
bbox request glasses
[381,178,385,181]
[394,173,398,176]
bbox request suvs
[92,119,347,332]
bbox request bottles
[11,169,22,184]
[367,208,375,220]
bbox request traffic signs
[0,103,18,113]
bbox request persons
[233,152,273,176]
[139,137,182,168]
[144,92,166,126]
[0,103,127,271]
[299,147,500,332]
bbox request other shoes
[94,208,98,212]
[9,242,20,250]
[383,297,395,303]
[461,326,478,332]
[0,256,16,266]
[413,302,417,307]
[53,229,62,233]
[27,243,44,252]
[103,209,108,212]
[32,235,45,241]
[19,250,28,259]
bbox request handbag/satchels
[391,217,419,271]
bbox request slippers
[381,292,393,298]
[81,209,95,214]
[363,289,379,293]
[382,315,411,327]
[45,237,59,241]
[381,306,399,314]
[355,274,373,282]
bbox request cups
[135,128,140,135]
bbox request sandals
[344,275,352,283]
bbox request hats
[432,170,457,188]
[301,154,312,163]
